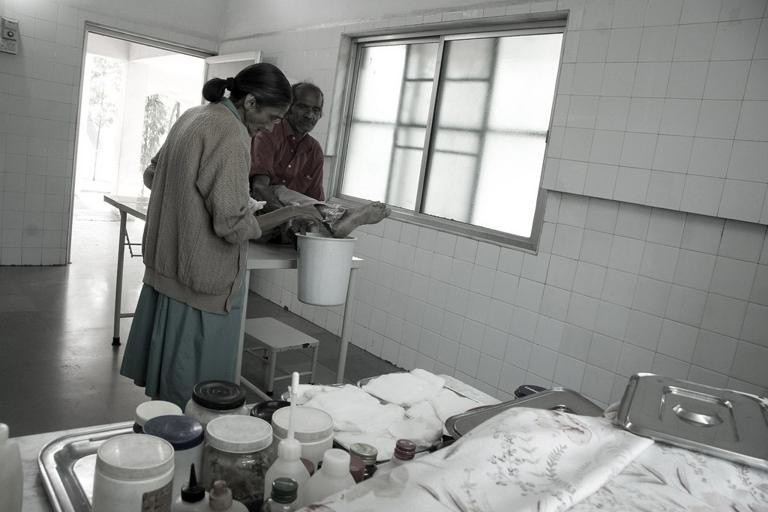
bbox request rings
[318,215,324,220]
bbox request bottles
[167,438,420,510]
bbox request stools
[245,316,319,392]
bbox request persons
[120,62,325,414]
[246,81,392,244]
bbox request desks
[7,374,503,512]
[103,194,364,400]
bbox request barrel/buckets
[294,230,358,306]
